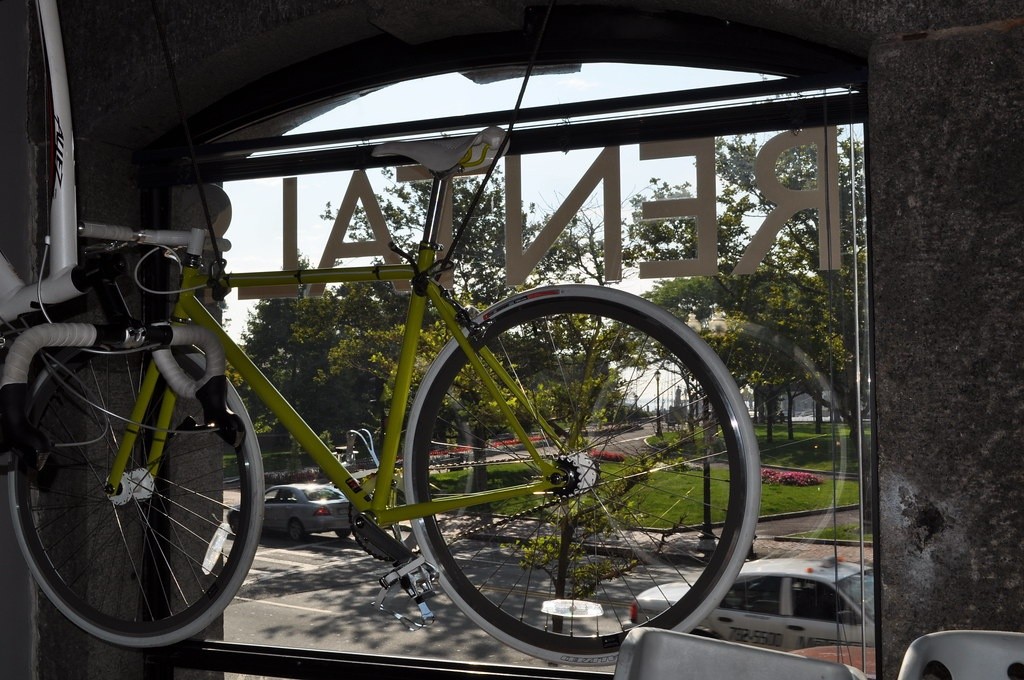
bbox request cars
[619,558,875,656]
[327,445,360,469]
[224,485,358,540]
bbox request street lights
[653,369,662,438]
[347,427,379,469]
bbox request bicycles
[0,124,760,669]
